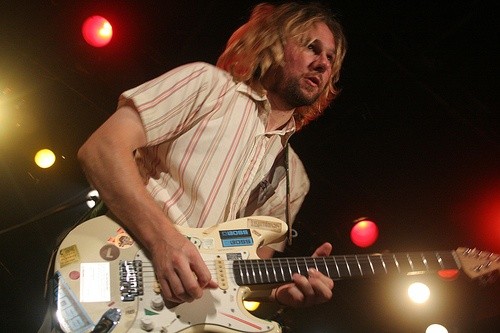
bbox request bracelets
[275,284,285,306]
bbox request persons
[79,2,348,306]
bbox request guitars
[51,215,500,333]
